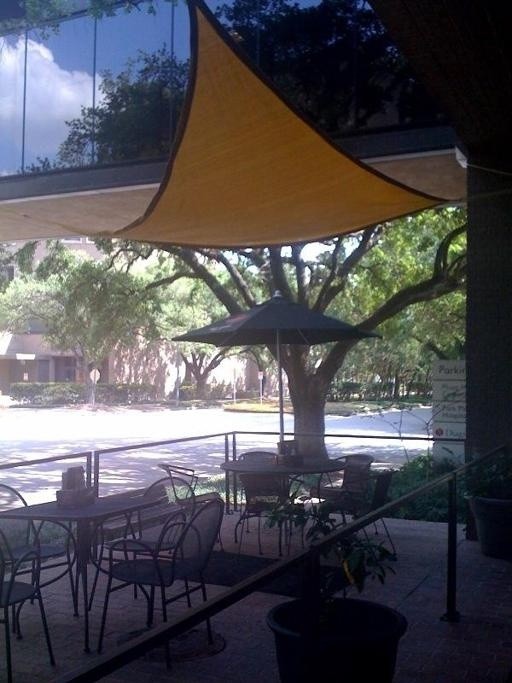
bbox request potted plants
[461,458,512,559]
[261,496,409,682]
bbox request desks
[0,496,161,653]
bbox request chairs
[158,463,225,554]
[0,526,57,683]
[87,477,196,613]
[0,483,80,634]
[220,452,401,557]
[97,498,224,679]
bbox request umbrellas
[172,287,383,466]
